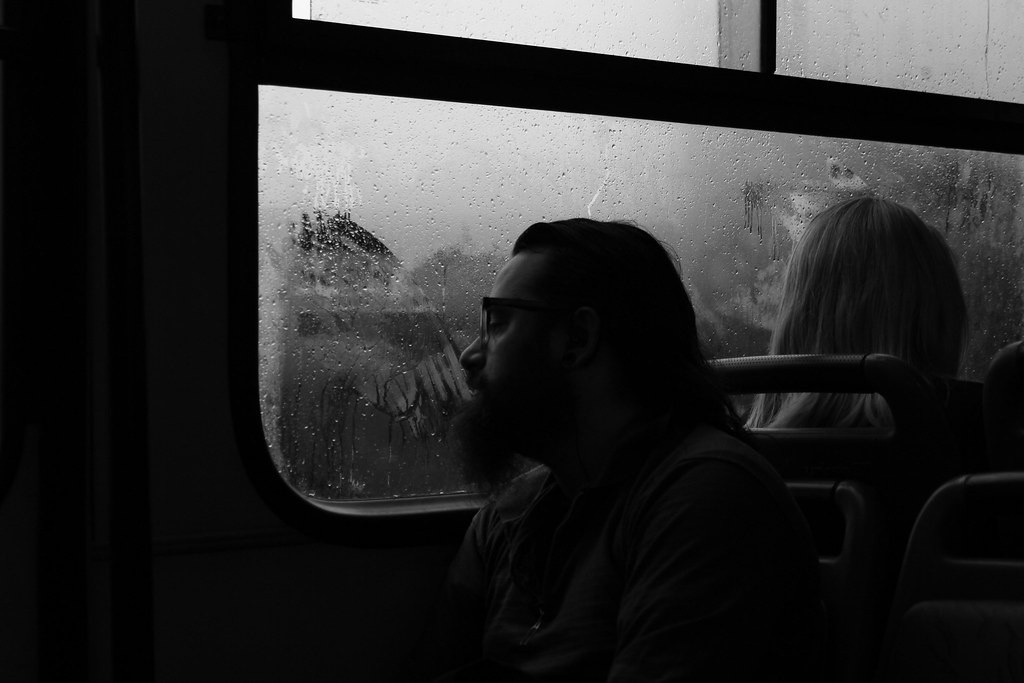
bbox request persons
[390,218,829,683]
[739,196,996,558]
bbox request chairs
[701,340,1024,683]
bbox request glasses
[478,296,624,350]
